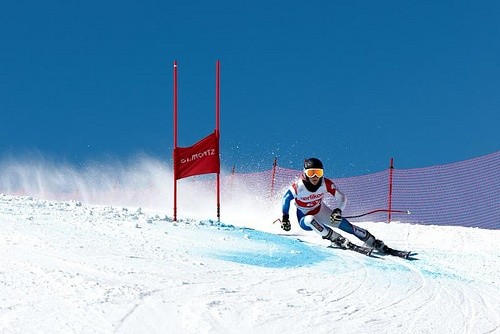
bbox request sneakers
[338,235,350,248]
[373,239,384,250]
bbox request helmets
[304,158,323,169]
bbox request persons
[279,157,412,259]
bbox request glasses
[304,168,323,178]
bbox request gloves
[330,208,343,224]
[282,220,291,231]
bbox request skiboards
[328,240,419,259]
